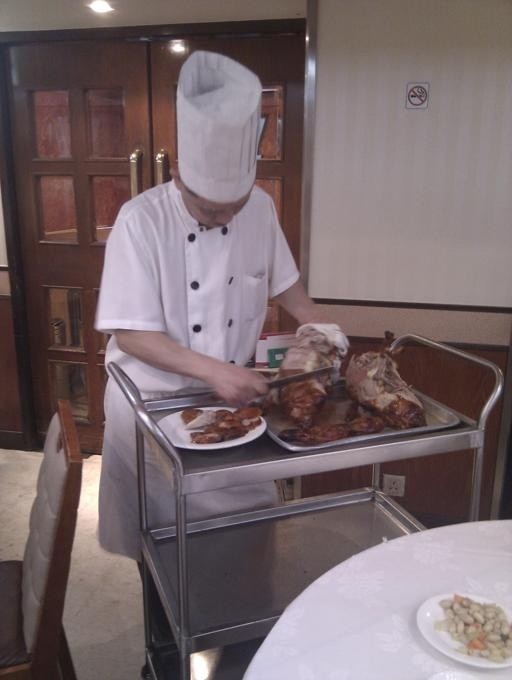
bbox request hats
[175,48,266,204]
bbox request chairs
[0,394,86,680]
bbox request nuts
[440,594,512,663]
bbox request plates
[154,405,271,453]
[414,587,512,670]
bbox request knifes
[212,364,337,401]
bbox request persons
[94,155,351,680]
